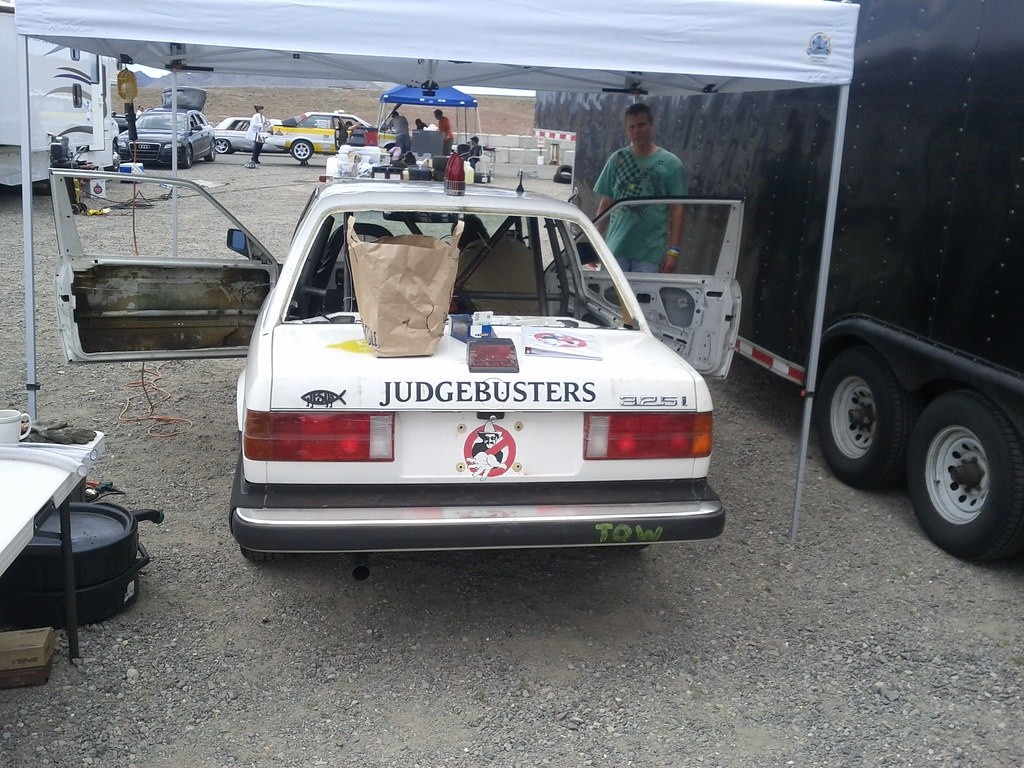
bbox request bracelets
[666,246,680,257]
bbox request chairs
[313,223,394,295]
[455,236,543,312]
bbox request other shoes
[252,159,261,164]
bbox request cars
[264,109,396,161]
[213,116,284,155]
[118,86,216,169]
[110,110,129,134]
[50,152,745,582]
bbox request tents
[14,1,861,561]
[377,85,478,143]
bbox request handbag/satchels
[347,216,465,358]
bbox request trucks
[0,5,120,187]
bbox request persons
[244,105,268,164]
[469,137,482,169]
[415,118,427,130]
[338,121,353,146]
[136,104,145,118]
[388,111,410,154]
[434,109,454,156]
[593,103,688,272]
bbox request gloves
[23,419,98,446]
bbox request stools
[371,166,403,179]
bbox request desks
[0,427,106,662]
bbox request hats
[470,137,479,143]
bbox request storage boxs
[79,179,106,199]
[0,626,55,672]
[119,163,143,183]
[0,655,54,690]
